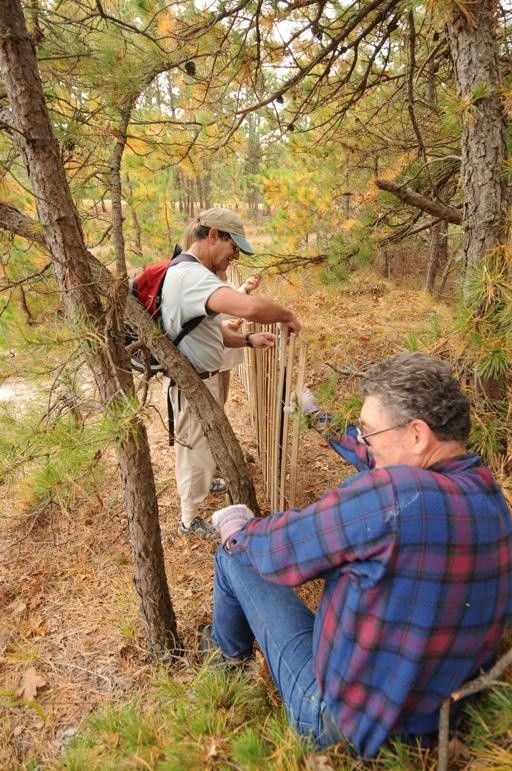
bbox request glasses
[356,419,412,447]
[222,234,240,252]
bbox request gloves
[283,385,320,419]
[212,504,254,552]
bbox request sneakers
[199,626,232,671]
[177,517,220,544]
[210,478,228,494]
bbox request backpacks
[126,254,205,373]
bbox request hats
[198,207,254,255]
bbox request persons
[198,351,512,766]
[159,207,302,540]
